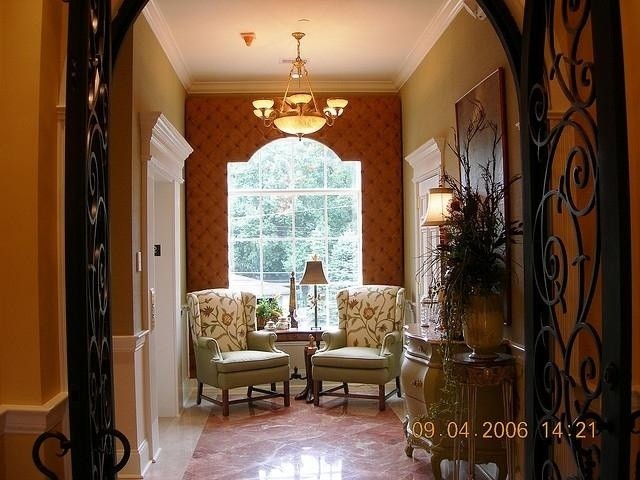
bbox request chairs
[311,285,406,411]
[187,288,291,417]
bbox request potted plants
[256,293,283,330]
[416,93,526,360]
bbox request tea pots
[276,316,291,330]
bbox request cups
[267,319,274,327]
[420,307,430,326]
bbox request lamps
[420,188,460,331]
[251,32,348,142]
[299,261,328,330]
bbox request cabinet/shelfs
[401,324,508,480]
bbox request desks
[452,352,514,480]
[275,331,332,404]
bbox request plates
[265,326,276,330]
